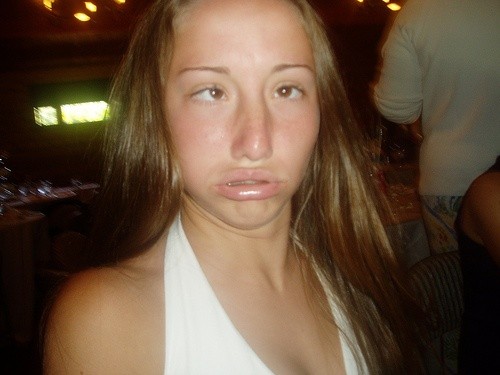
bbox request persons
[455,153,500,375]
[38,1,409,375]
[362,0,500,259]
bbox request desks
[0,183,106,295]
